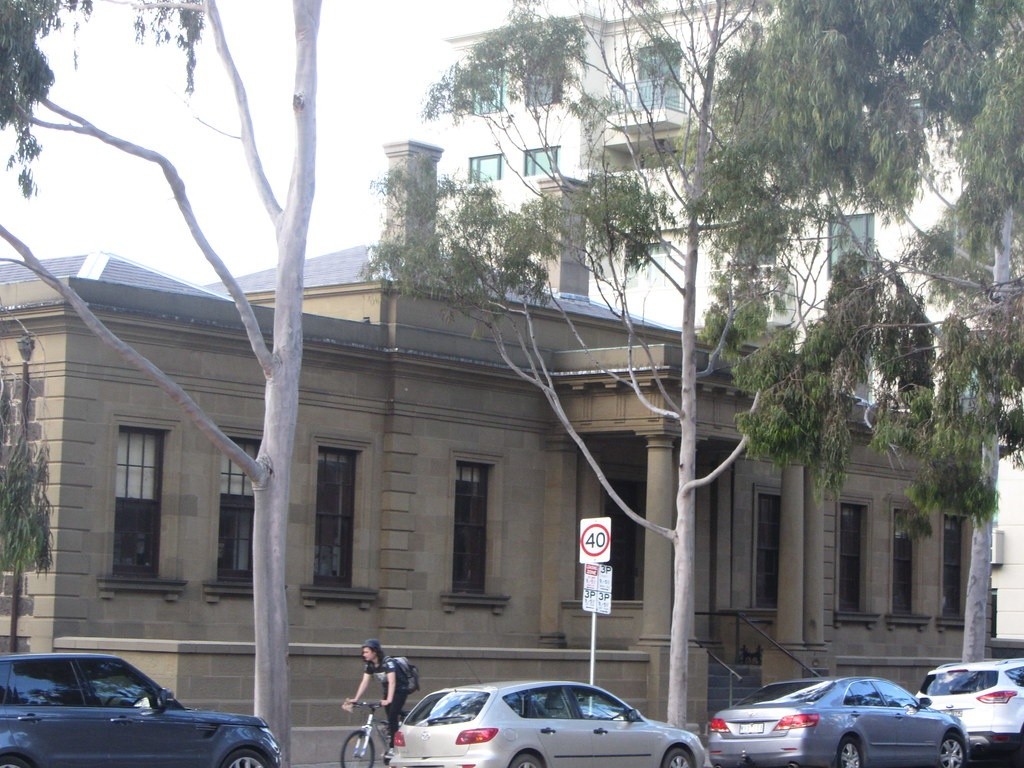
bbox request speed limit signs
[582,524,609,556]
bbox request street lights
[10,336,35,653]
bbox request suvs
[914,658,1024,759]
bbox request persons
[346,639,410,759]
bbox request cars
[390,680,705,768]
[709,676,970,768]
[0,653,283,768]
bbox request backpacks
[393,656,420,695]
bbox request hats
[362,639,381,651]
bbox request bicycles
[340,701,411,768]
